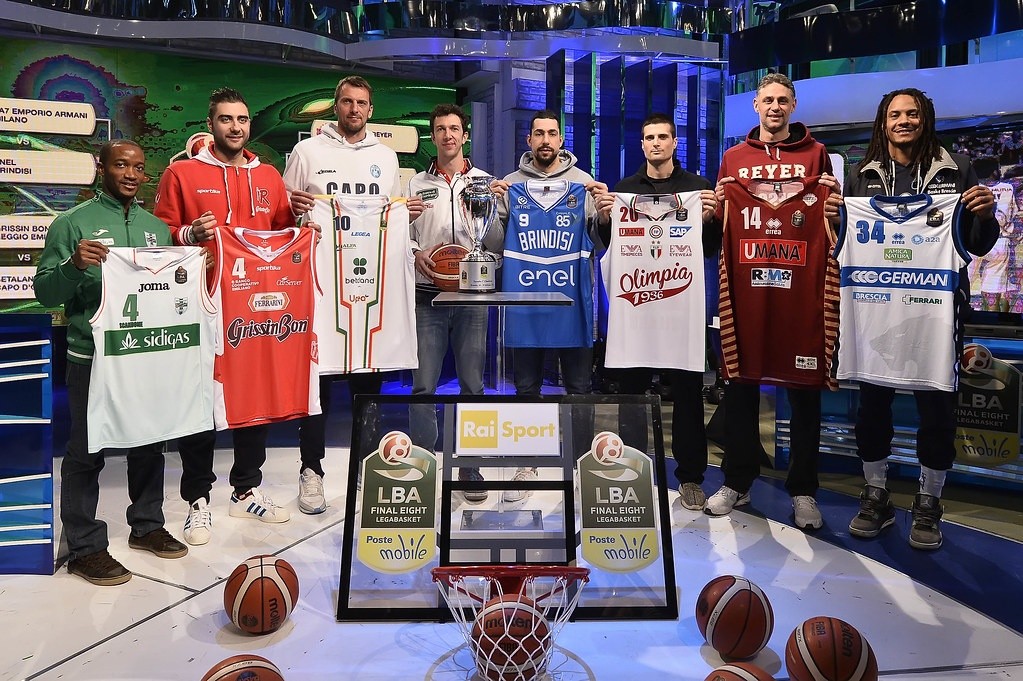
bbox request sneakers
[228,486,290,524]
[704,485,751,516]
[297,468,327,515]
[127,524,188,558]
[848,481,898,537]
[679,483,706,511]
[68,548,133,586]
[905,491,945,550]
[182,497,213,546]
[790,495,823,531]
[502,466,539,502]
[458,466,488,500]
[352,479,364,515]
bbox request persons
[406,106,504,500]
[590,116,723,511]
[32,137,215,585]
[951,128,1023,311]
[153,88,322,546]
[283,76,424,514]
[703,73,842,527]
[482,110,608,502]
[824,88,1000,548]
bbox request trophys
[457,176,497,288]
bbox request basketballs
[785,615,880,681]
[695,574,775,659]
[222,554,299,634]
[426,243,471,290]
[703,661,776,681]
[200,653,285,681]
[469,593,550,681]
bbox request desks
[432,292,574,529]
